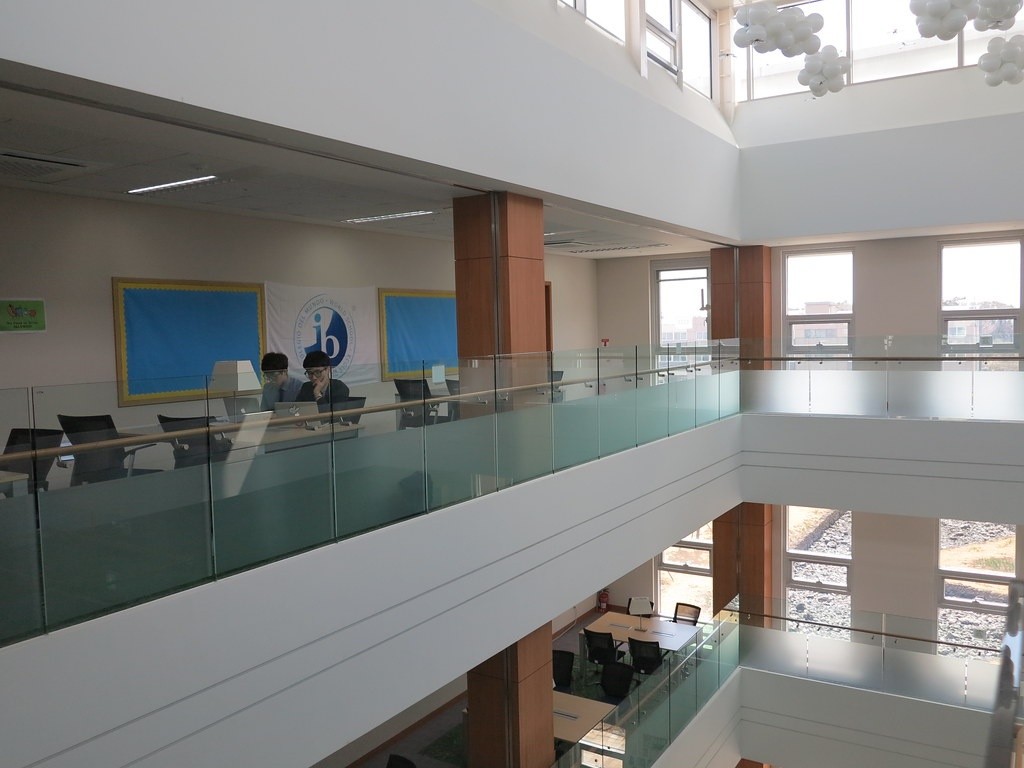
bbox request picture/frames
[111,277,268,409]
[378,287,459,381]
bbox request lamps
[629,597,653,631]
[207,360,263,424]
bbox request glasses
[263,371,283,381]
[304,367,328,378]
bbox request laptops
[273,402,329,428]
[223,397,261,423]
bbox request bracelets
[314,393,321,399]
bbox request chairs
[56,414,158,487]
[673,603,701,626]
[347,397,367,423]
[393,371,565,430]
[0,428,64,494]
[386,754,417,768]
[626,598,655,618]
[156,415,232,470]
[552,627,671,725]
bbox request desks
[0,471,29,498]
[462,689,619,761]
[119,422,366,452]
[579,611,703,690]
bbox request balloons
[733,2,824,58]
[798,45,850,97]
[909,0,979,41]
[974,0,1024,31]
[978,35,1024,87]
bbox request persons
[260,352,303,411]
[295,351,349,424]
